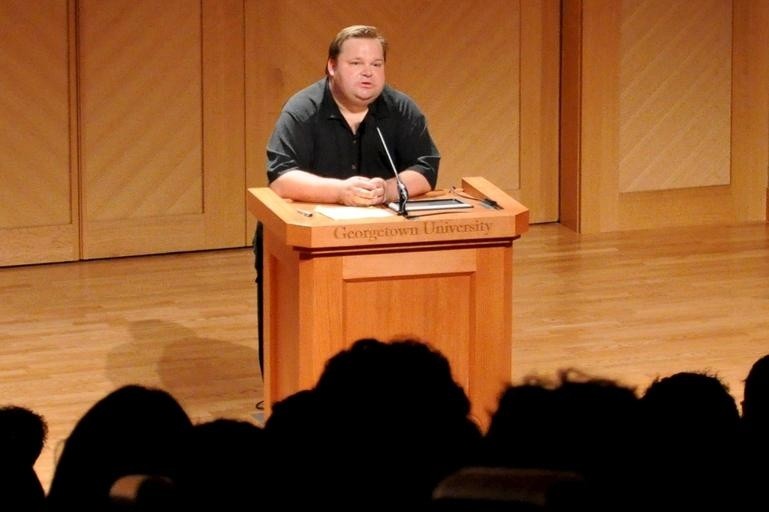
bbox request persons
[253,24,442,411]
[1,335,483,510]
[434,355,768,512]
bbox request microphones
[365,106,411,216]
